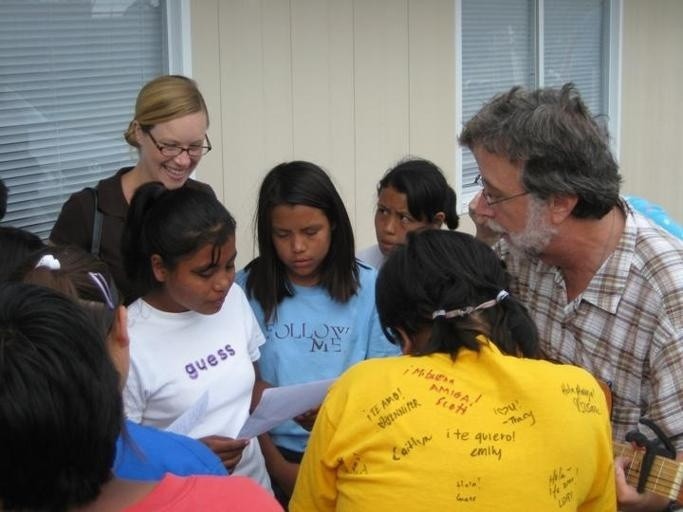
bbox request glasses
[147,131,212,158]
[475,174,532,208]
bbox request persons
[48,74,217,307]
[10,244,230,481]
[121,181,323,498]
[352,160,459,272]
[0,283,286,512]
[0,226,46,289]
[289,227,618,511]
[458,82,683,512]
[468,189,683,247]
[234,161,404,512]
